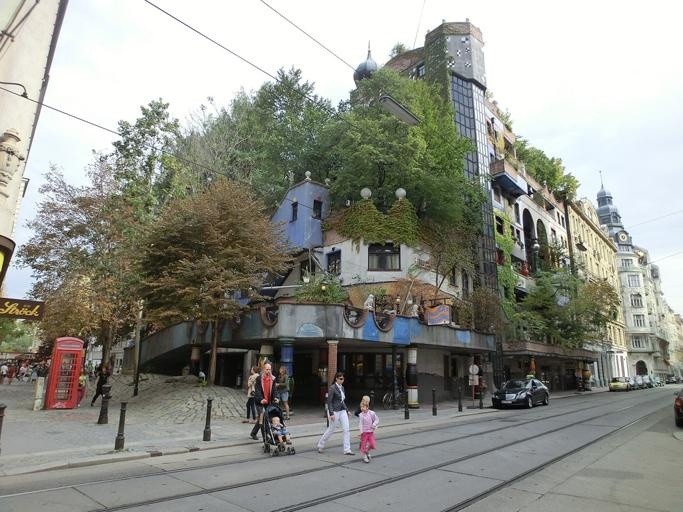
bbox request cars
[491,378,550,408]
[608,375,680,391]
[674,388,682,427]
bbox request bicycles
[382,393,407,410]
[362,390,376,409]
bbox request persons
[0,355,110,406]
[241,357,379,462]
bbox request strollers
[260,399,295,457]
